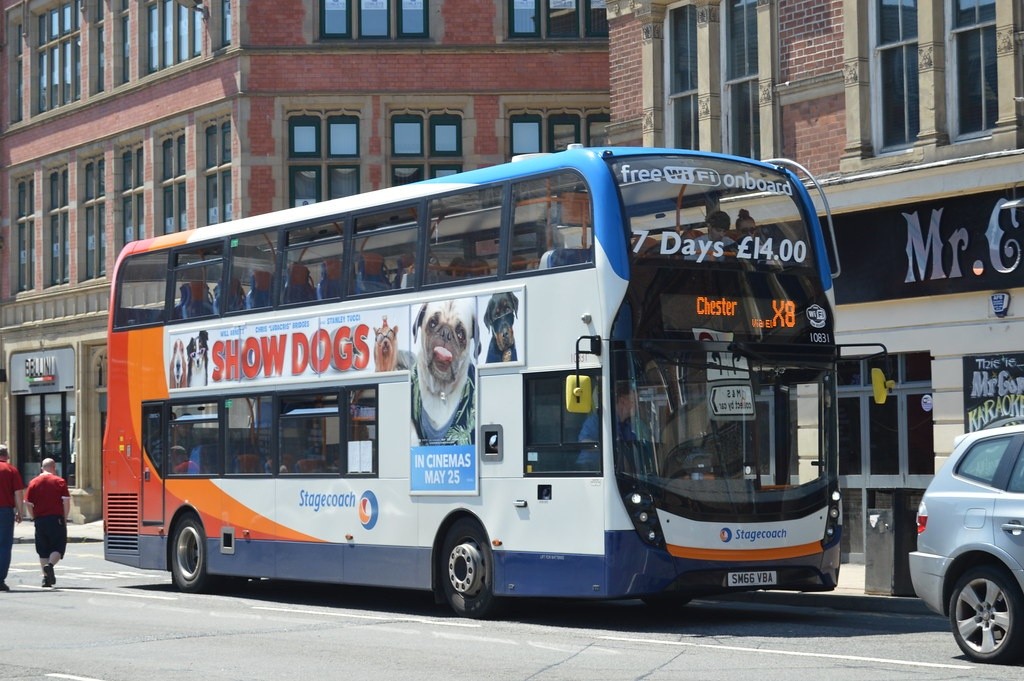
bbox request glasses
[738,227,755,234]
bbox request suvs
[909,416,1024,663]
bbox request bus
[101,144,899,625]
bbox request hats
[170,446,187,456]
[0,445,9,455]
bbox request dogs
[413,301,476,447]
[484,290,518,363]
[169,331,208,390]
[373,326,399,373]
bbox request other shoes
[42,579,51,587]
[43,564,56,584]
[0,583,9,590]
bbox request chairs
[173,451,345,475]
[172,227,754,326]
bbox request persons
[170,413,200,474]
[302,201,308,205]
[576,380,646,472]
[0,444,70,591]
[685,208,775,263]
[394,241,489,288]
[537,231,568,270]
